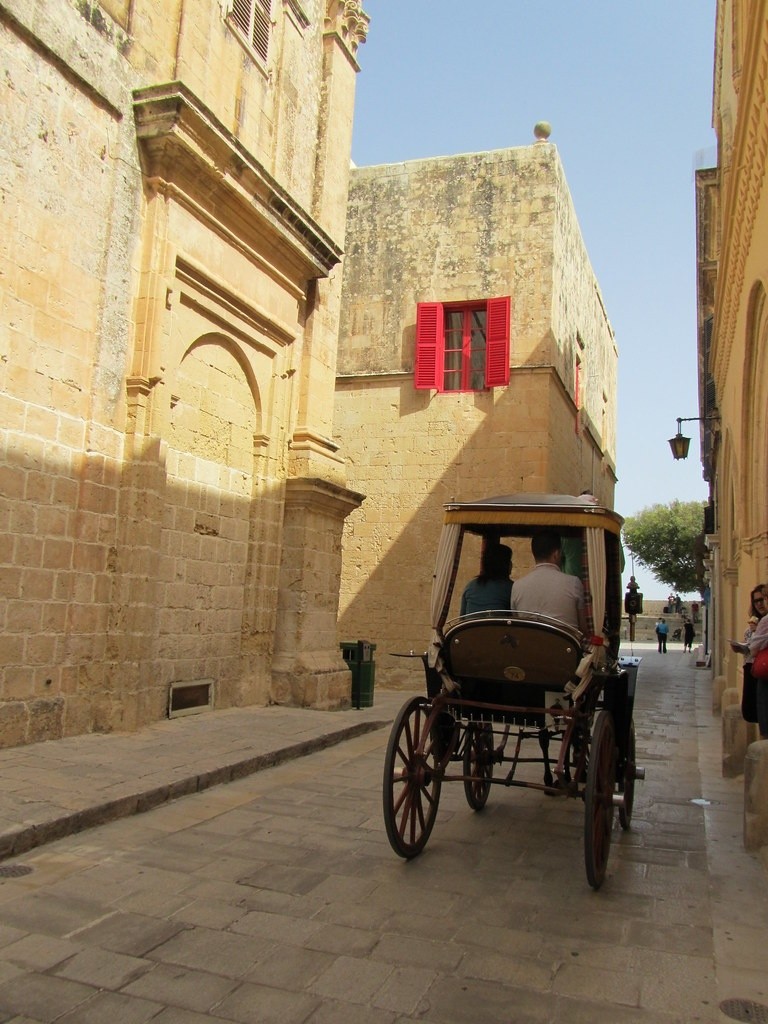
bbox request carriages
[382,492,646,891]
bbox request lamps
[668,418,691,459]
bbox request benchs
[441,608,586,708]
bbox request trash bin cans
[339,640,377,708]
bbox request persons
[730,584,768,740]
[682,619,695,653]
[550,698,563,732]
[459,544,512,619]
[655,618,669,654]
[681,605,686,617]
[511,532,589,635]
[691,601,699,621]
[559,536,625,583]
[668,593,675,611]
[674,594,680,613]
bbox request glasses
[751,597,765,604]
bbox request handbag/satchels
[655,627,660,634]
[751,648,768,677]
[689,631,696,637]
